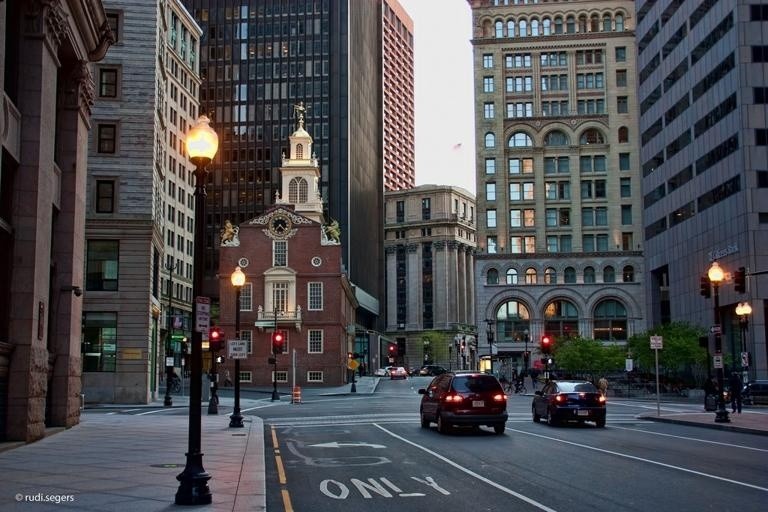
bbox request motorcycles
[171,375,181,393]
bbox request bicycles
[503,381,528,396]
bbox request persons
[323,222,340,245]
[171,368,181,389]
[221,220,235,244]
[510,368,521,394]
[703,376,718,408]
[596,376,609,396]
[729,371,743,414]
[223,367,234,386]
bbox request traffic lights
[348,352,352,358]
[542,336,550,354]
[733,267,746,296]
[273,332,283,354]
[389,345,394,352]
[218,331,225,350]
[208,327,220,352]
[699,273,710,300]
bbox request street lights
[230,266,246,428]
[270,307,284,401]
[524,328,529,376]
[448,341,451,371]
[487,321,495,373]
[423,340,429,365]
[735,302,744,352]
[174,113,221,509]
[743,301,752,353]
[708,261,730,423]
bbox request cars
[420,365,448,376]
[374,365,393,377]
[531,379,607,428]
[391,366,407,379]
[409,368,420,377]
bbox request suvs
[418,372,509,433]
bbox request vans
[741,380,768,406]
